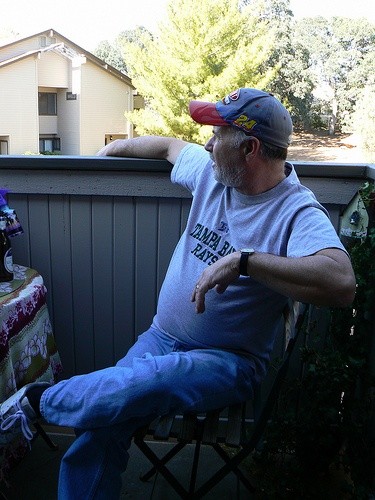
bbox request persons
[0,88,357,500]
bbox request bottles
[0,217,15,282]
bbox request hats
[188,88,292,149]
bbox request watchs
[239,248,255,276]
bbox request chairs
[135,297,307,500]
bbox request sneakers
[0,382,51,450]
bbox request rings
[196,284,200,289]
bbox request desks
[0,264,64,486]
